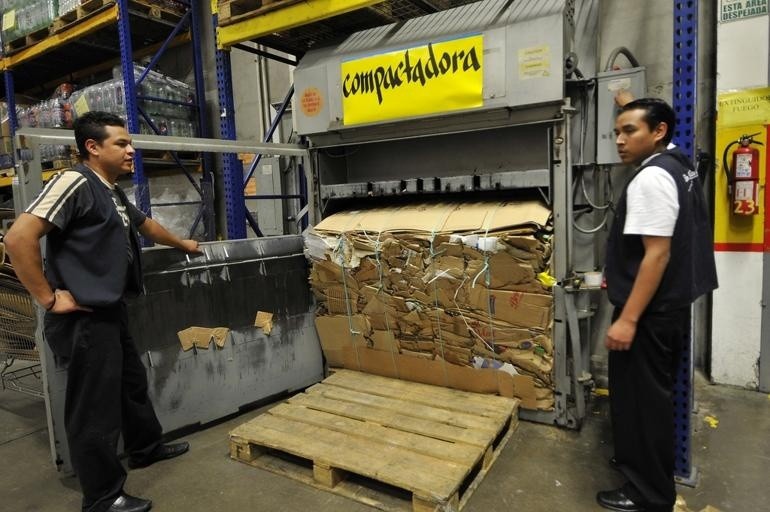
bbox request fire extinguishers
[723,132,764,217]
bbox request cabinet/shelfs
[0,0,220,281]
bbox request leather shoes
[107,493,153,512]
[127,441,190,470]
[596,455,640,511]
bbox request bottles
[16,62,198,162]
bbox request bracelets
[46,293,56,312]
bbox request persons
[597,89,719,512]
[3,112,205,512]
